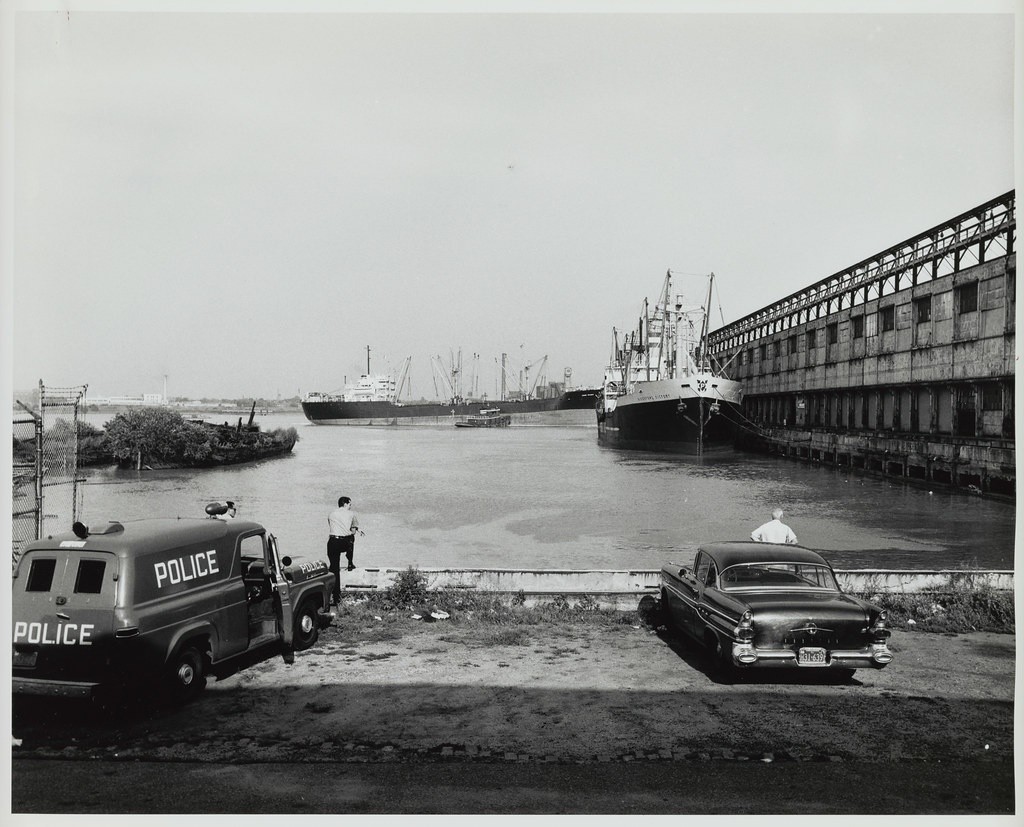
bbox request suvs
[13,502,336,712]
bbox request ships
[300,342,601,426]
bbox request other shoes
[330,598,340,606]
[348,562,355,571]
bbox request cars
[660,541,893,673]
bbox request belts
[330,535,352,539]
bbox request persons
[326,497,365,606]
[750,507,798,571]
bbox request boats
[594,267,746,457]
[454,407,511,427]
